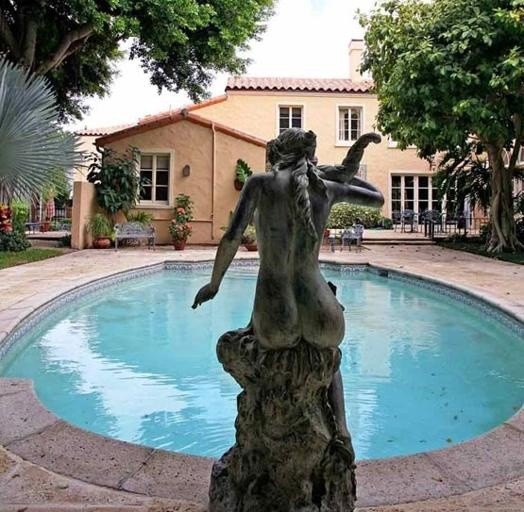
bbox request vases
[173,237,187,250]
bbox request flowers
[169,208,191,240]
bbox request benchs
[26,222,36,234]
[114,224,156,251]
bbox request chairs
[343,224,363,247]
[393,208,442,233]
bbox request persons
[189,125,386,462]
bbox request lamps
[183,165,190,176]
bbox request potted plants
[84,212,114,248]
[242,224,258,251]
